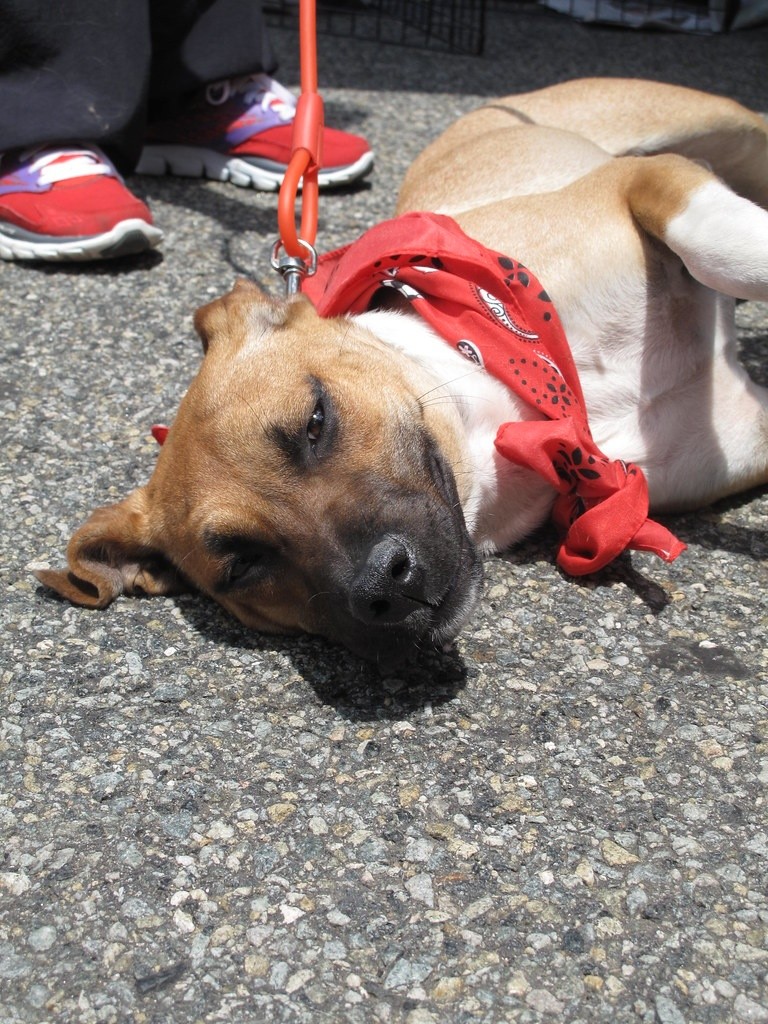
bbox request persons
[0,0,374,262]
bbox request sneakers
[0,142,161,264]
[132,70,373,191]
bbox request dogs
[30,76,768,663]
